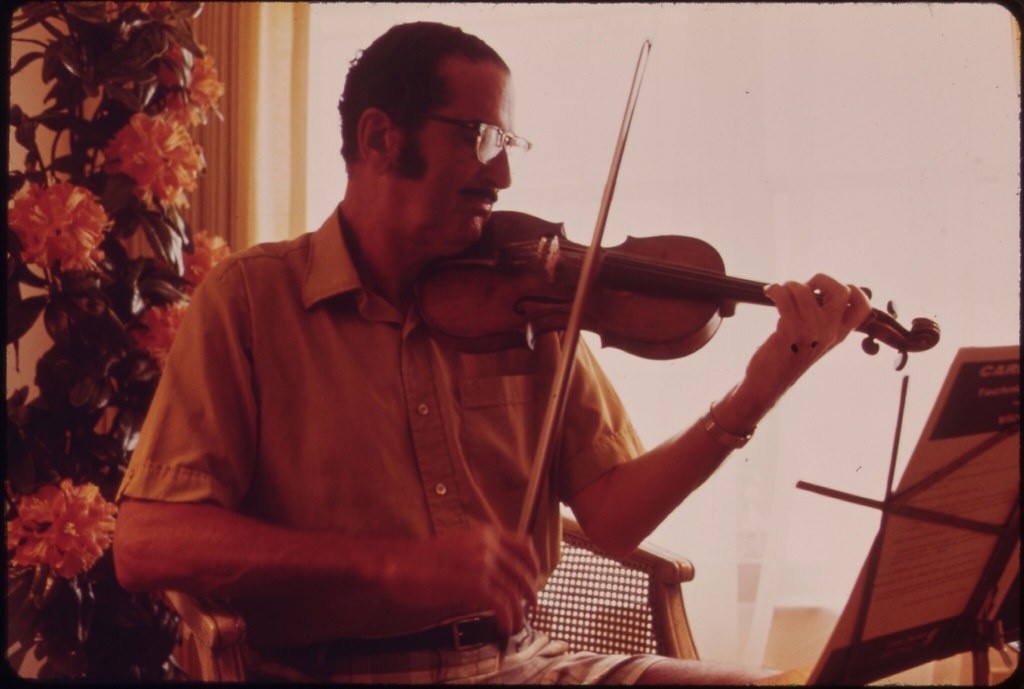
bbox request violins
[416,210,943,361]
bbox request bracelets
[699,399,755,449]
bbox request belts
[327,616,497,650]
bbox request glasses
[420,114,533,173]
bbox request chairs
[157,511,700,689]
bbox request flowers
[0,0,233,689]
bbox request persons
[111,19,874,689]
